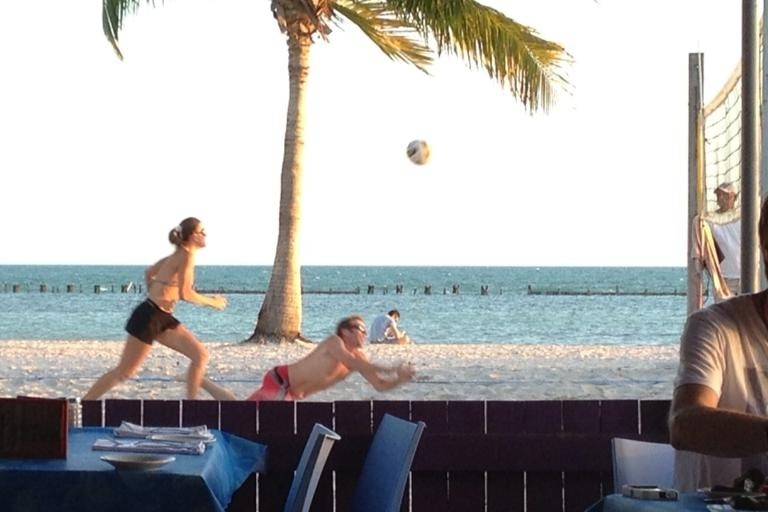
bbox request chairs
[282,411,426,512]
[609,436,698,494]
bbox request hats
[714,183,740,195]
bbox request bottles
[67,396,85,430]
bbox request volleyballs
[408,140,430,164]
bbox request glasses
[196,228,206,237]
[349,323,367,334]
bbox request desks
[0,427,225,511]
[601,492,768,511]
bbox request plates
[99,453,177,469]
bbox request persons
[200,316,419,401]
[668,193,767,495]
[703,181,741,293]
[368,310,409,345]
[81,217,227,400]
